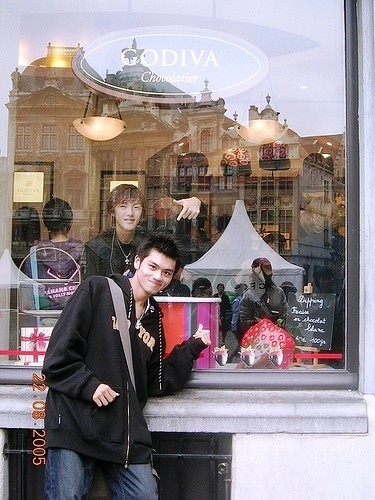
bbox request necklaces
[109,229,135,278]
[133,297,150,330]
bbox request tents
[180,198,306,296]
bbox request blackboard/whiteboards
[284,291,337,350]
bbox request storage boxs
[20,327,54,363]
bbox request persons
[152,257,344,363]
[83,183,209,279]
[287,197,346,369]
[24,197,88,312]
[40,234,212,500]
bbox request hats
[191,277,211,290]
[251,258,273,275]
[278,281,297,293]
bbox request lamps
[72,90,127,142]
[234,77,288,145]
